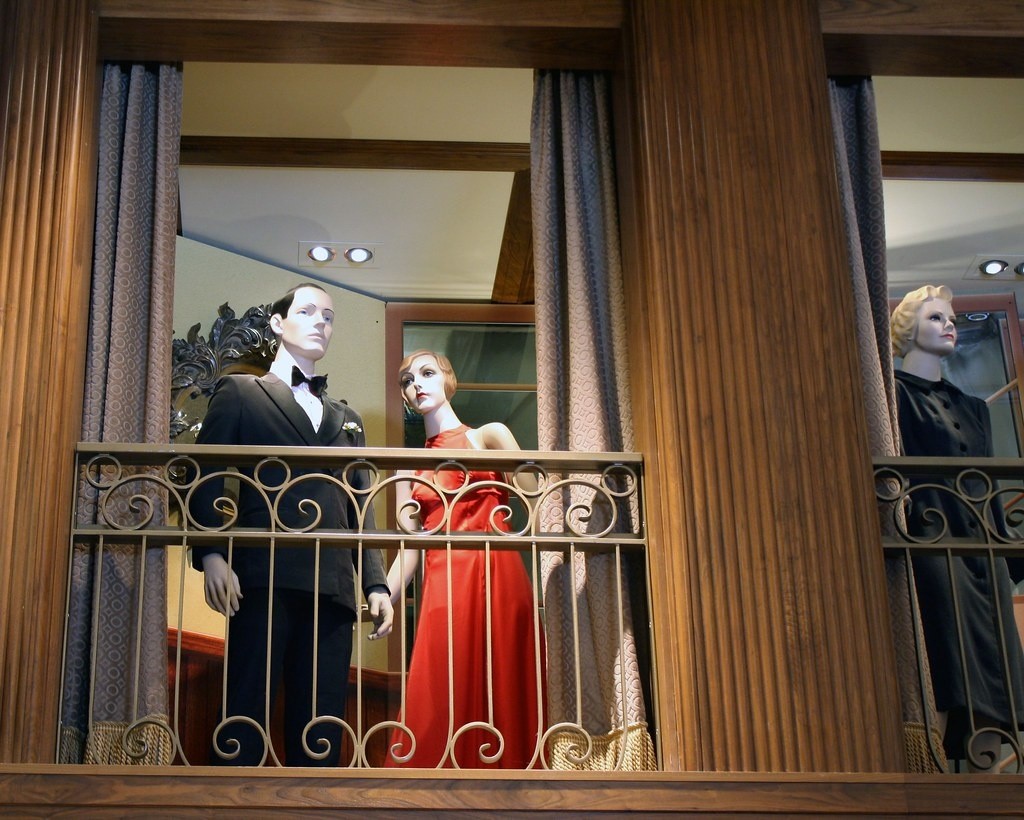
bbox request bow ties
[290,368,329,398]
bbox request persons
[889,283,1024,772]
[186,285,395,766]
[382,350,551,770]
